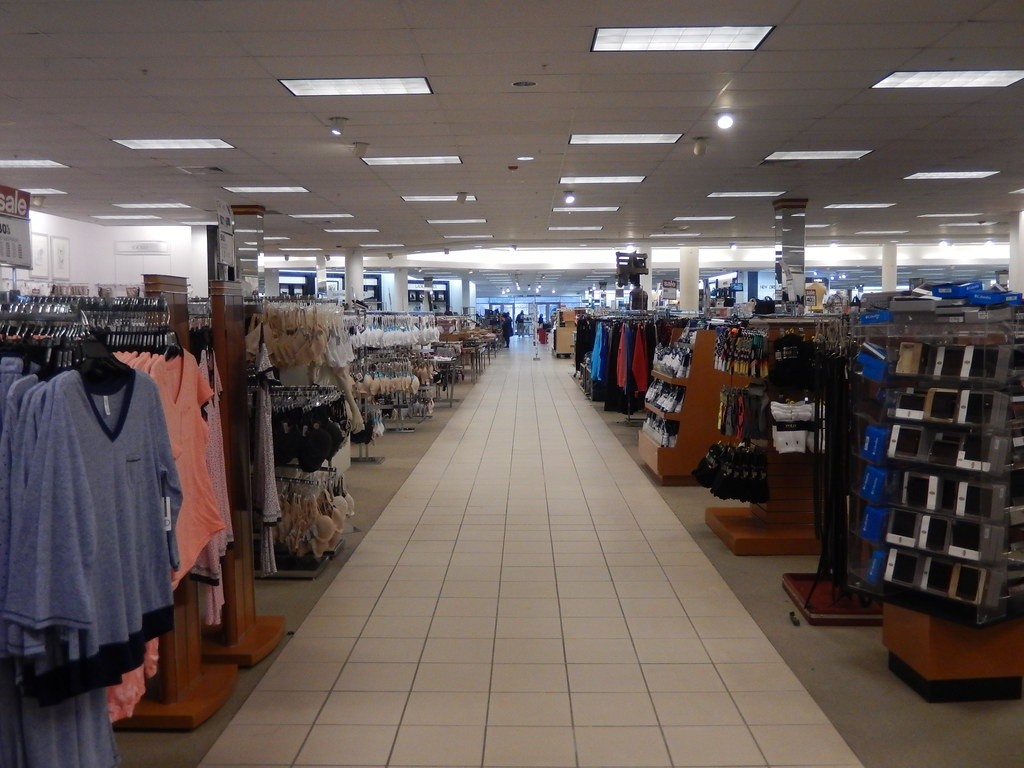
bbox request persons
[539,314,543,323]
[501,312,513,348]
[332,366,364,434]
[516,310,525,338]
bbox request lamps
[444,248,449,254]
[692,138,707,157]
[33,195,44,207]
[325,255,330,261]
[285,255,289,260]
[329,116,349,136]
[457,192,467,203]
[387,253,393,259]
[715,112,734,130]
[354,143,369,157]
[563,190,576,204]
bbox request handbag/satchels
[745,297,774,314]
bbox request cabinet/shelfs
[847,303,1024,703]
[639,318,819,561]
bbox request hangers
[244,290,338,306]
[594,318,671,325]
[343,311,497,406]
[247,384,344,413]
[0,295,213,379]
[811,316,849,359]
[276,463,343,491]
